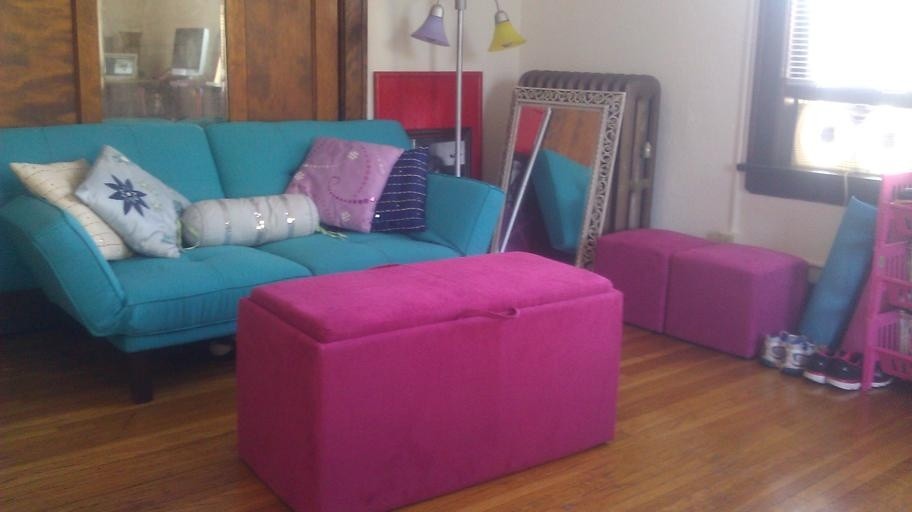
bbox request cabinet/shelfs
[856,172,912,390]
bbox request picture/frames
[488,84,627,275]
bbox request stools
[592,224,808,360]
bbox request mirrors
[96,1,230,123]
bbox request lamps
[408,1,529,175]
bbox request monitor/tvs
[170,27,210,77]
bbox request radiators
[517,69,660,237]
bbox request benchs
[230,251,625,511]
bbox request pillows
[9,132,428,257]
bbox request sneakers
[760,331,802,369]
[781,340,834,376]
[803,347,834,384]
[827,350,895,391]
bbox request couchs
[0,116,510,407]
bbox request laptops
[103,52,139,81]
